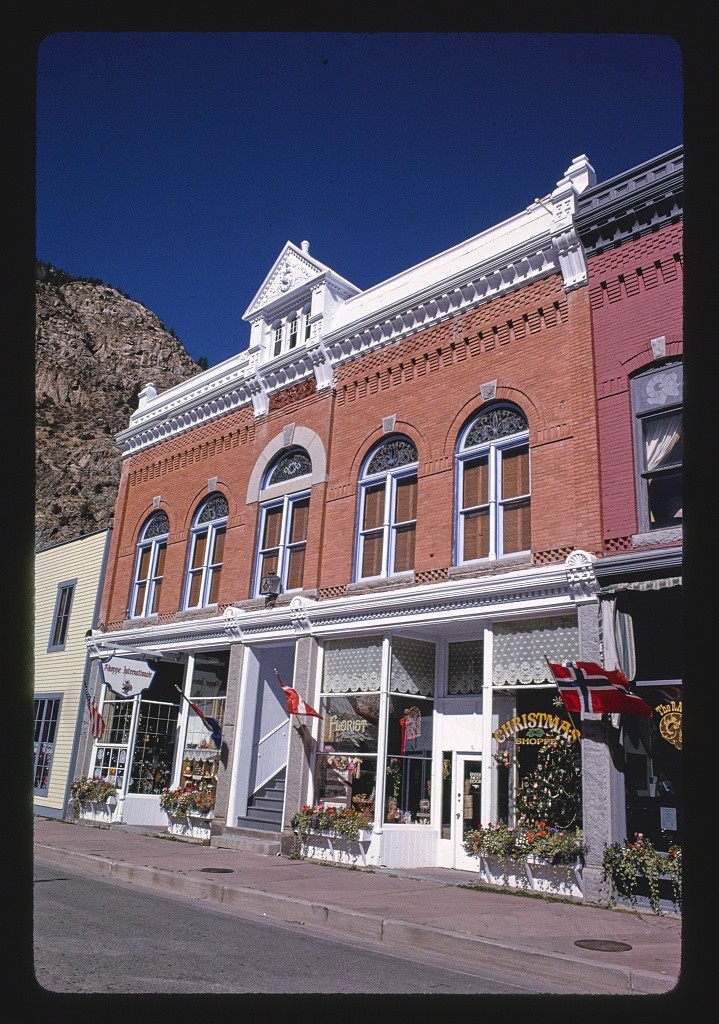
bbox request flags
[549,661,652,717]
[187,699,222,746]
[280,680,323,719]
[84,686,105,739]
